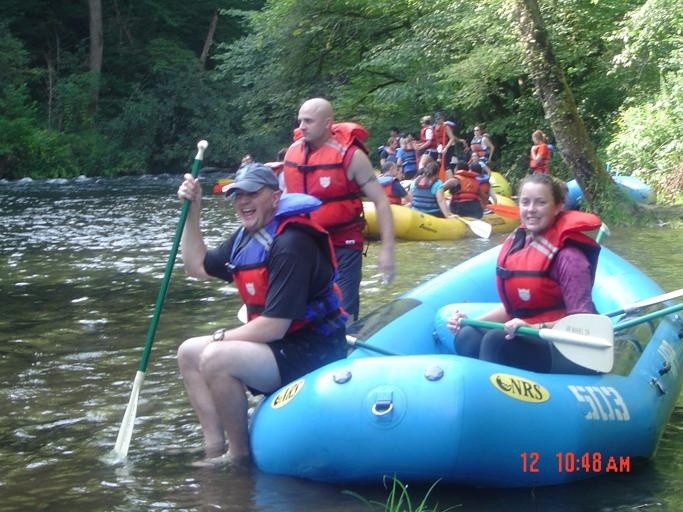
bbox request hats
[222,162,280,197]
[435,112,444,119]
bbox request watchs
[212,327,227,343]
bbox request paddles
[457,314,615,374]
[609,289,682,318]
[113,139,211,460]
[456,216,493,238]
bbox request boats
[563,174,657,210]
[246,224,683,491]
[489,171,514,198]
[361,193,521,244]
[213,177,234,195]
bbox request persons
[376,114,496,218]
[174,164,346,467]
[239,154,253,164]
[528,130,549,176]
[444,175,600,377]
[276,147,289,194]
[265,97,395,325]
